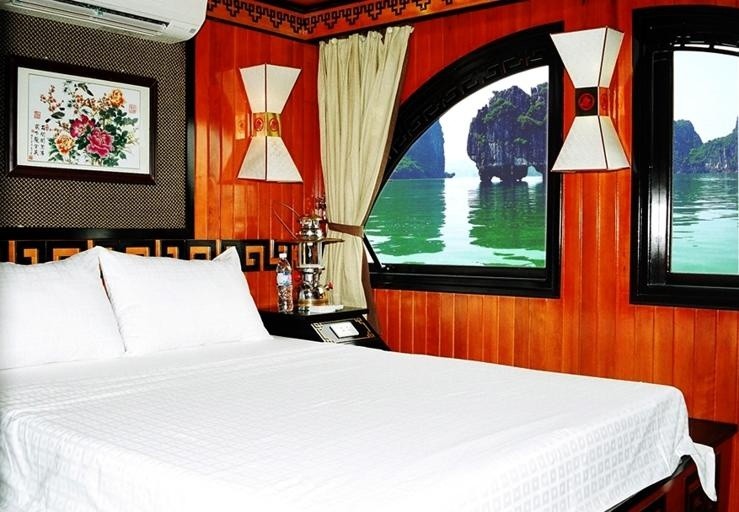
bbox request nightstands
[257,299,390,350]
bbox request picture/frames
[4,53,159,186]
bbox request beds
[1,221,737,511]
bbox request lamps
[546,26,631,175]
[238,60,309,185]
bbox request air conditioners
[6,1,207,46]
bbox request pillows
[1,245,125,373]
[98,242,273,361]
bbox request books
[309,304,344,310]
[311,310,335,312]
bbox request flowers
[33,79,140,167]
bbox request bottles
[276,253,294,312]
